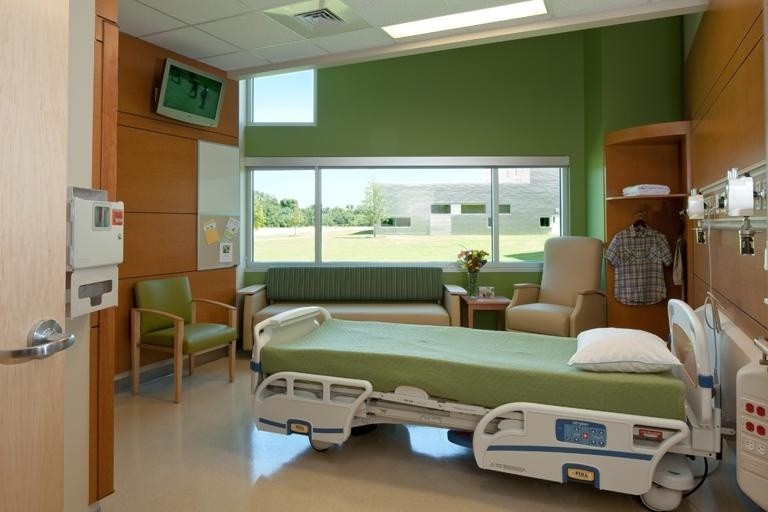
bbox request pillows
[566,325,684,375]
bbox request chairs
[130,274,239,403]
[504,235,606,338]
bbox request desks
[462,295,512,329]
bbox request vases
[464,272,481,299]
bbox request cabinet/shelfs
[604,119,696,338]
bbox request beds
[253,297,718,424]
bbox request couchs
[236,265,468,353]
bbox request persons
[200,85,208,109]
[190,77,200,99]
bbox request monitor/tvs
[150,55,225,127]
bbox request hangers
[631,210,649,230]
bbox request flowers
[457,246,490,295]
